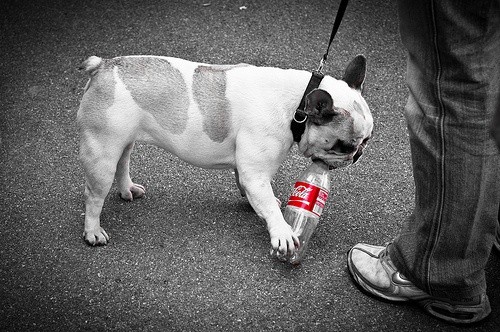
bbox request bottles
[269,160,331,264]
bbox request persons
[346,0,500,324]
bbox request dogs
[76,54,374,256]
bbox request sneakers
[347,243,491,323]
